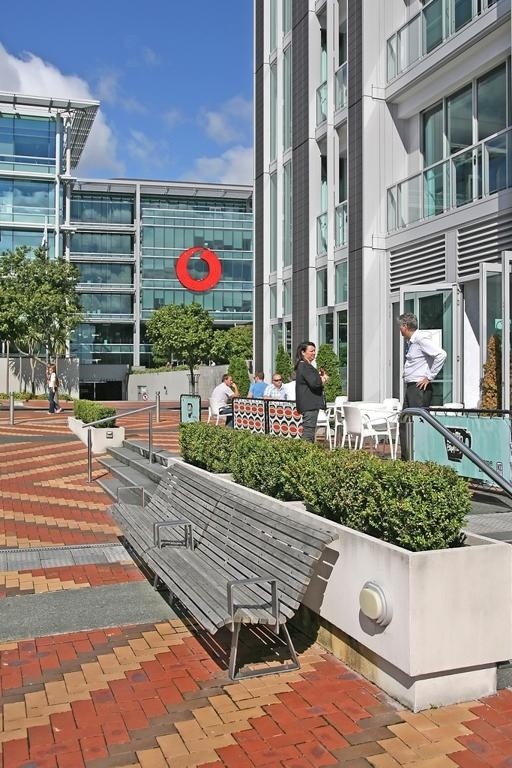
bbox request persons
[209,372,239,428]
[45,363,65,415]
[246,370,267,400]
[163,385,168,401]
[283,367,328,401]
[292,341,329,443]
[398,311,448,461]
[261,373,288,401]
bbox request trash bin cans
[137,385,147,401]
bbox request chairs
[206,396,232,427]
[316,396,462,459]
